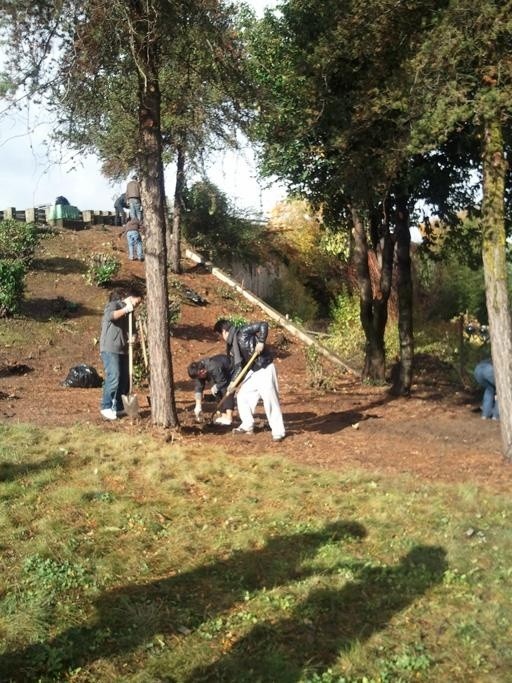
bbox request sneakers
[215,415,282,442]
[100,407,126,420]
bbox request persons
[125,218,144,262]
[125,175,142,222]
[186,353,244,425]
[99,288,144,420]
[212,318,286,441]
[113,193,130,226]
[473,359,499,421]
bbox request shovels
[203,351,258,427]
[121,302,139,417]
[138,314,151,407]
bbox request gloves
[227,381,237,393]
[211,384,220,397]
[194,403,202,417]
[255,341,264,355]
[125,303,134,312]
